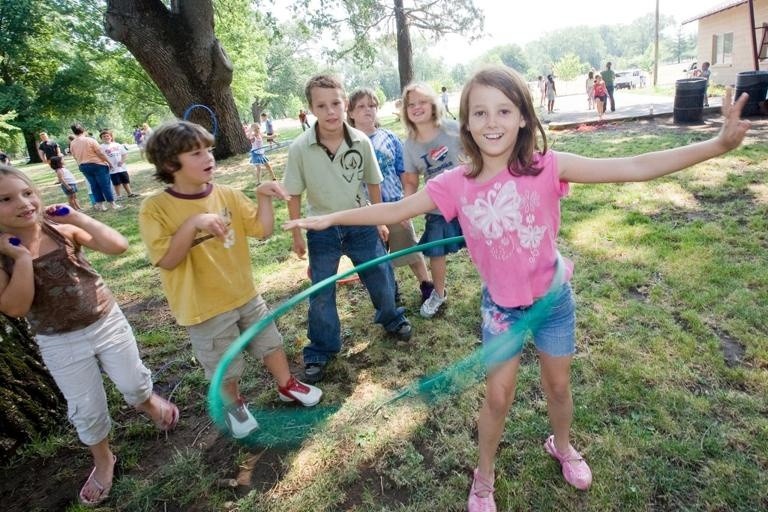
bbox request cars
[614,70,648,90]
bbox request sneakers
[94,194,139,213]
[420,282,448,317]
[469,468,496,512]
[393,284,402,307]
[396,322,412,337]
[277,377,324,406]
[304,361,328,380]
[543,434,592,490]
[225,400,259,439]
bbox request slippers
[80,454,116,505]
[144,394,180,429]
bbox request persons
[347,87,435,307]
[537,63,621,115]
[299,110,310,132]
[137,121,324,439]
[697,62,711,106]
[250,124,276,186]
[441,87,456,120]
[0,165,179,507]
[401,83,467,319]
[0,122,154,212]
[282,75,413,385]
[261,113,281,150]
[281,64,752,512]
